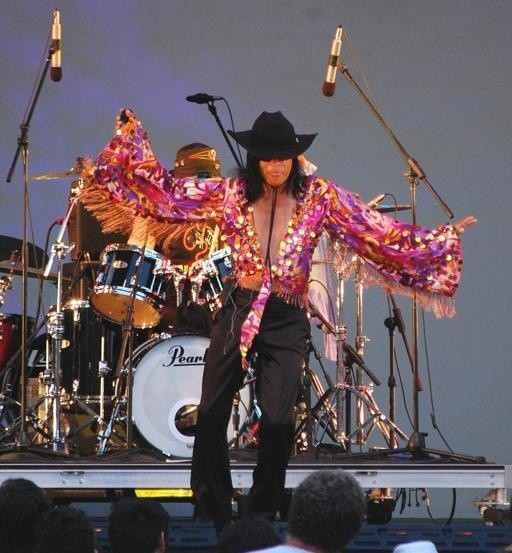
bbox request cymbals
[26,171,90,179]
[0,260,72,282]
[375,205,414,212]
[1,235,49,268]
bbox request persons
[246,466,366,553]
[214,517,282,552]
[94,104,481,527]
[1,478,52,553]
[108,499,172,553]
[32,506,100,553]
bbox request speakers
[68,182,126,260]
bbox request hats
[227,111,318,160]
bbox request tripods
[66,318,133,451]
[0,35,77,459]
[292,247,410,458]
[0,249,137,458]
[339,62,487,464]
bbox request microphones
[322,25,342,97]
[51,10,62,81]
[186,93,221,104]
[368,194,385,208]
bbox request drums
[186,248,235,322]
[49,302,131,404]
[89,244,170,329]
[117,333,253,461]
